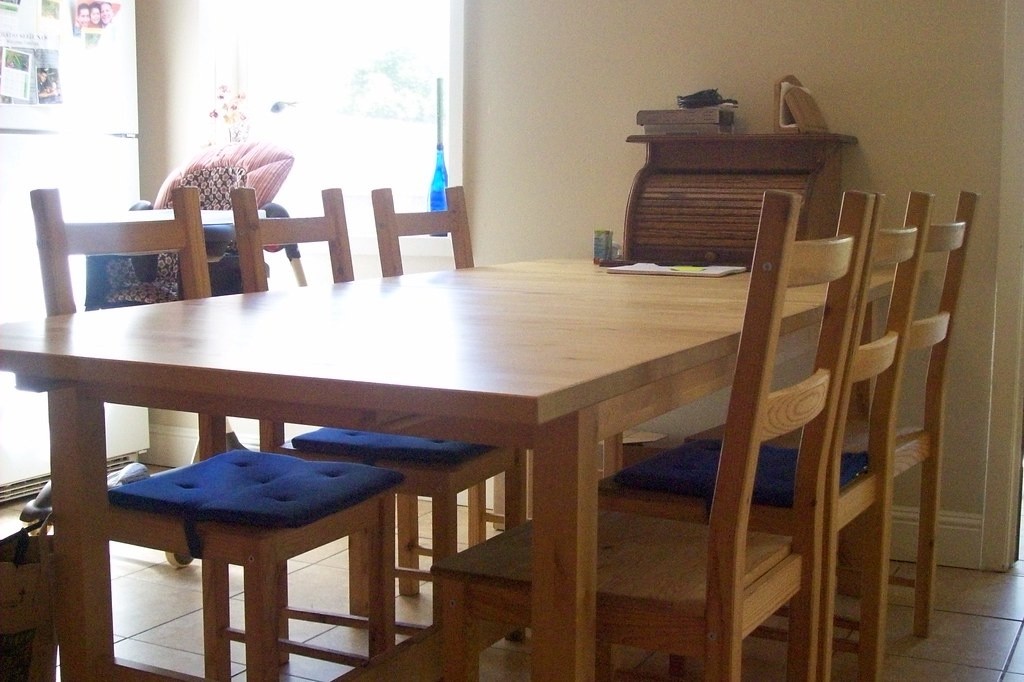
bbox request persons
[37,69,59,104]
[76,3,113,29]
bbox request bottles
[428,145,449,237]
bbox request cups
[594,230,612,262]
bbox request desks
[1,253,932,682]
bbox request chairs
[370,189,622,595]
[431,190,875,682]
[596,188,979,682]
[229,187,526,670]
[29,188,404,681]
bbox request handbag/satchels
[0,511,60,682]
